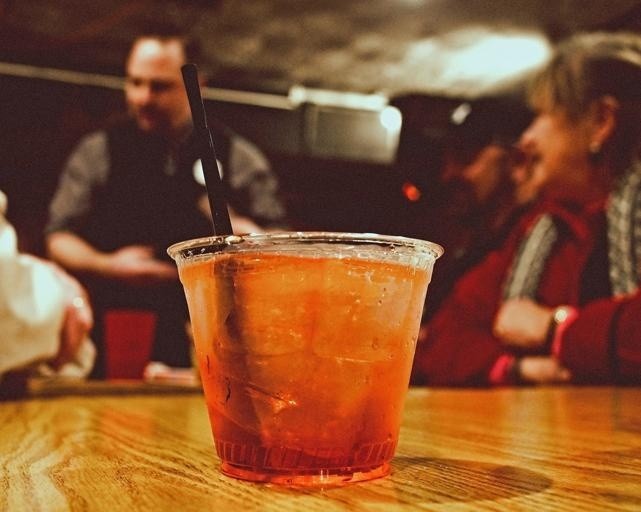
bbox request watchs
[541,302,573,357]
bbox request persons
[471,28,640,388]
[0,189,100,380]
[41,22,289,371]
[402,89,541,388]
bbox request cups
[168,232,443,490]
[104,308,158,385]
